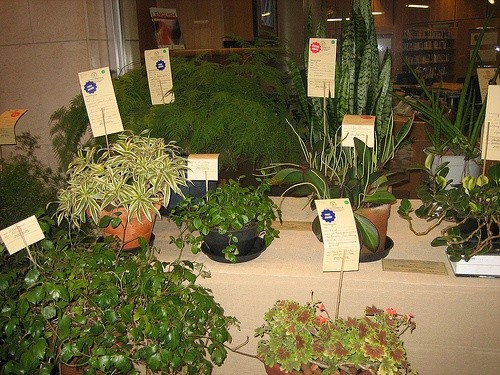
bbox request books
[402,22,457,76]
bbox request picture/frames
[252,0,278,40]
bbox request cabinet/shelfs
[392,24,454,82]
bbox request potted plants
[0,206,240,375]
[44,126,192,252]
[396,152,500,281]
[169,181,287,267]
[251,294,418,375]
[387,1,500,194]
[263,117,415,270]
